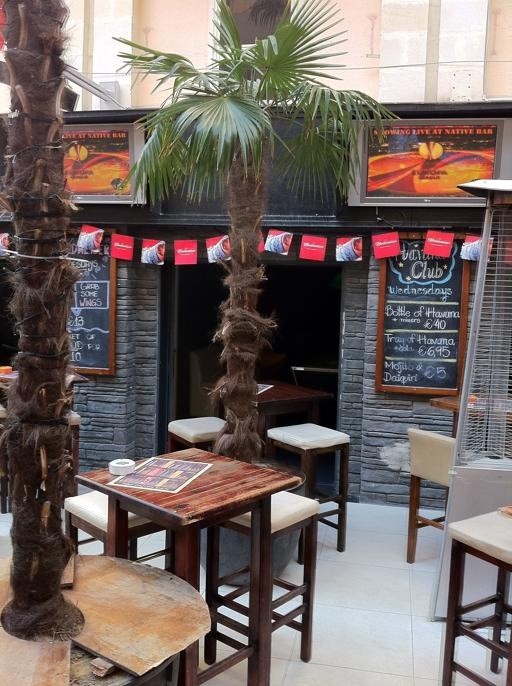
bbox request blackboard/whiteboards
[65,227,117,376]
[375,232,471,396]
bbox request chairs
[403,423,459,565]
[187,349,225,419]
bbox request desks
[72,446,302,685]
[218,376,334,475]
[428,395,512,519]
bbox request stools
[60,490,175,578]
[0,402,83,518]
[201,488,322,683]
[165,414,229,457]
[442,500,511,686]
[263,418,353,566]
